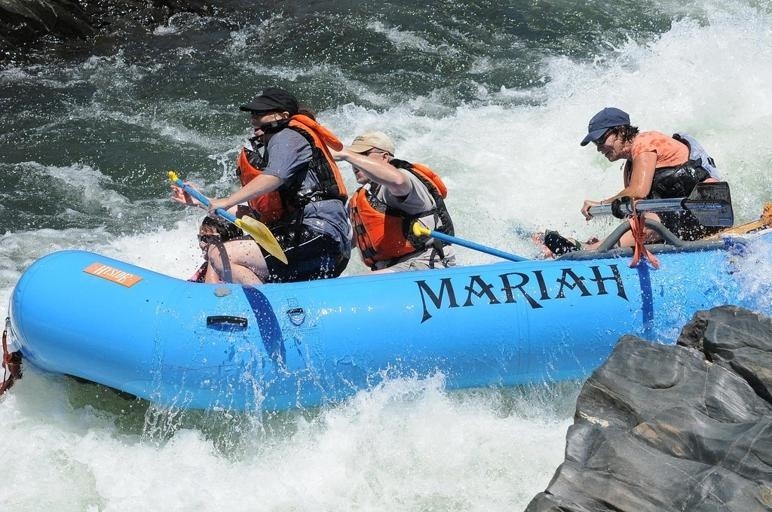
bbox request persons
[330,129,456,276]
[169,80,349,288]
[185,214,242,285]
[532,106,732,263]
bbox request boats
[8,226,772,413]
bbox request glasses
[592,126,618,145]
[250,110,276,120]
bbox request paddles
[588,182,734,227]
[168,169,288,265]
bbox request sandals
[543,229,582,257]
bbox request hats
[580,107,630,148]
[345,130,395,158]
[238,87,299,115]
